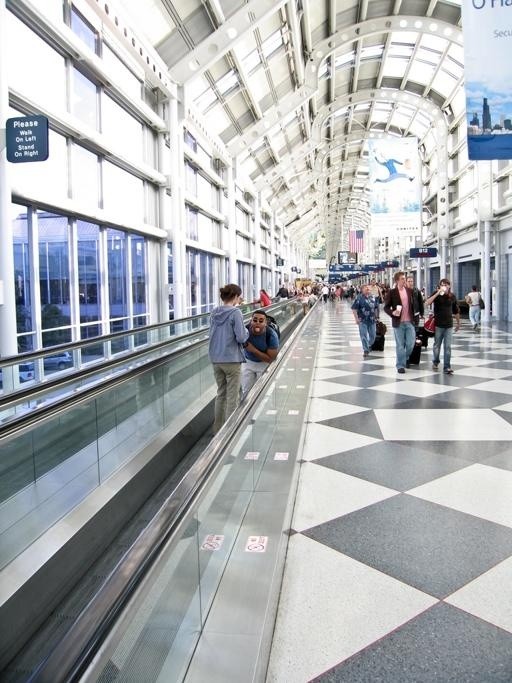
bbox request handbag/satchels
[376,321,386,335]
[479,299,485,309]
[424,316,435,338]
[375,296,381,304]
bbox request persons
[423,277,460,373]
[350,283,380,356]
[258,275,429,319]
[207,283,253,435]
[464,284,481,330]
[240,309,280,401]
[383,271,422,373]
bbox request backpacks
[246,316,280,347]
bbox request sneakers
[432,363,453,374]
[472,323,481,331]
[363,347,372,357]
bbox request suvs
[44,351,72,370]
[0,361,35,384]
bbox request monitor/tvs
[338,251,357,264]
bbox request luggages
[371,334,384,351]
[409,337,421,364]
[415,317,428,347]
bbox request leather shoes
[398,360,409,373]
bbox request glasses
[253,319,263,323]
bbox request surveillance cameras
[309,53,313,61]
[441,106,449,112]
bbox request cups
[396,305,402,315]
[440,286,447,297]
